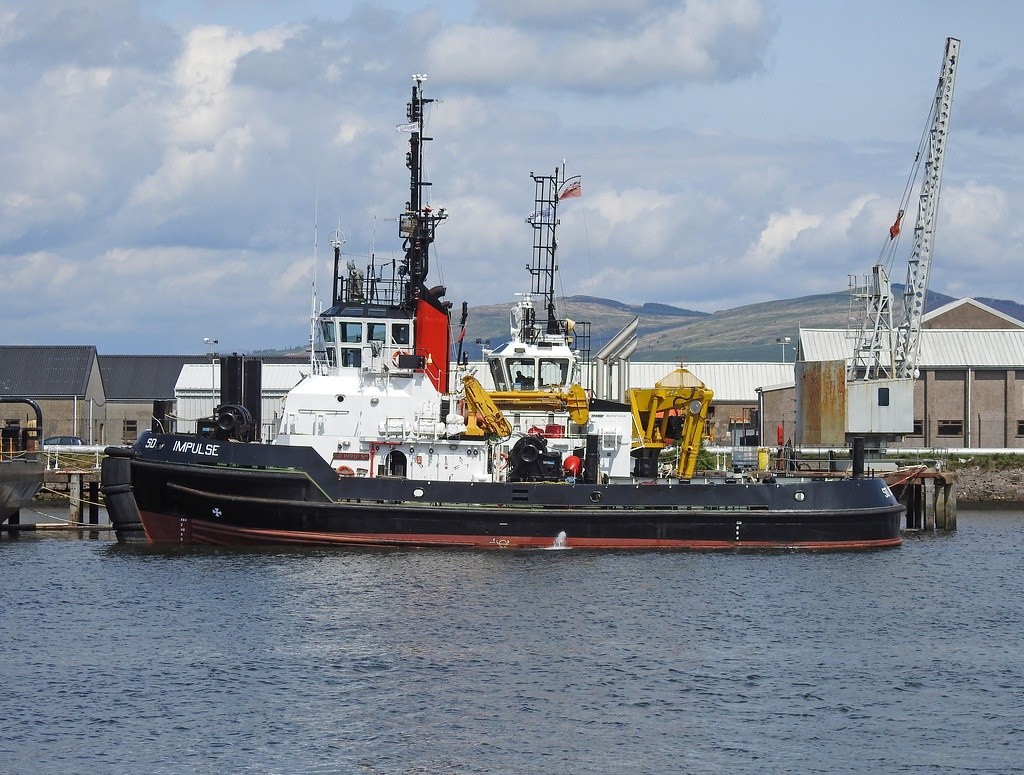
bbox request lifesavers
[392,350,408,368]
[337,465,354,477]
[493,453,509,470]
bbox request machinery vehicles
[844,37,959,432]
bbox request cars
[44,435,86,446]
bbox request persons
[515,371,526,383]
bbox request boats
[0,396,46,524]
[97,73,907,556]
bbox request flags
[395,121,418,133]
[560,181,582,200]
